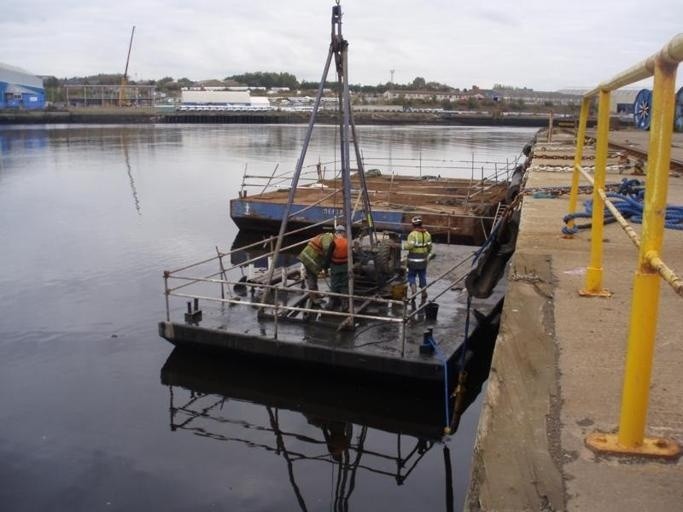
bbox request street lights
[390,68,394,90]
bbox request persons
[295,233,333,305]
[391,215,431,301]
[325,225,349,311]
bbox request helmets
[335,224,346,230]
[412,216,423,225]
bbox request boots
[309,289,324,304]
[411,284,427,298]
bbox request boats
[154,0,521,435]
[230,149,519,245]
[231,230,302,267]
[160,346,492,512]
